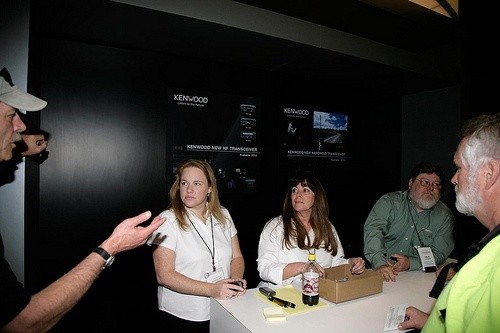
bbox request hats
[0,66,47,111]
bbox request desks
[210,259,457,333]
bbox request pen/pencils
[268,296,296,308]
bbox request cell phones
[259,286,276,296]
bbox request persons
[398,115,500,333]
[0,75,167,333]
[363,169,456,283]
[146,160,247,333]
[255,176,365,287]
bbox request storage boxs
[318,264,383,305]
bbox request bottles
[301,254,320,306]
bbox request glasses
[416,178,442,189]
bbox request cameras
[228,281,243,292]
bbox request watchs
[92,247,115,268]
